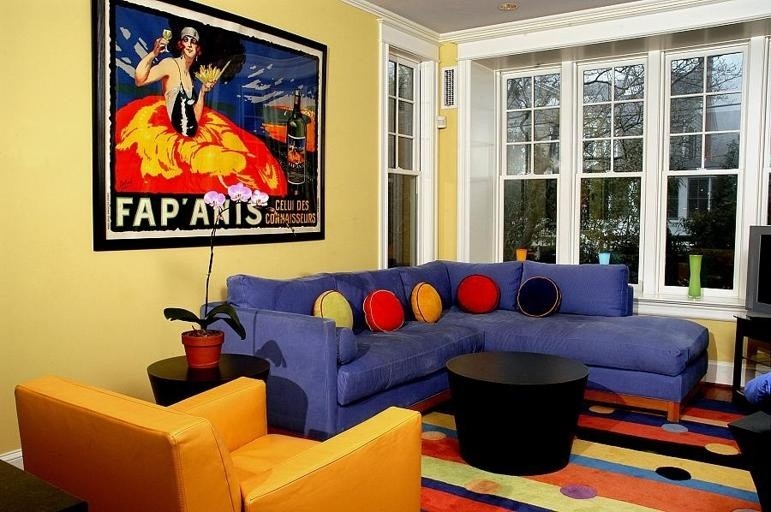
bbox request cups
[161,30,172,54]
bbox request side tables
[146,355,269,407]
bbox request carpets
[420,398,762,512]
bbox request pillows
[336,326,358,365]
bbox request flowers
[163,182,270,341]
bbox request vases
[688,253,702,299]
[516,249,526,259]
[597,255,613,264]
[182,331,224,368]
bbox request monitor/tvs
[745,226,771,318]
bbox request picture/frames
[90,0,327,252]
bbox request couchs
[201,260,710,441]
[13,376,420,512]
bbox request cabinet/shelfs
[732,315,771,399]
